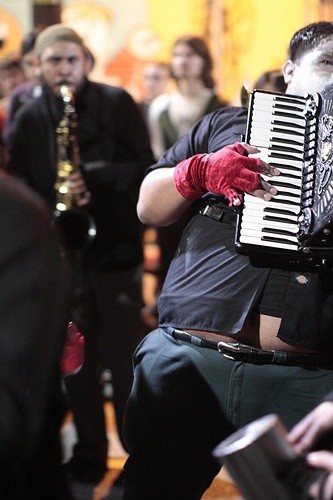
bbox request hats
[35,24,83,58]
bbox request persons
[146,36,230,319]
[0,31,43,173]
[135,60,171,122]
[4,23,156,485]
[286,388,333,500]
[0,172,75,500]
[123,21,333,500]
[253,69,288,93]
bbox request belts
[163,326,332,368]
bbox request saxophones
[52,83,99,258]
